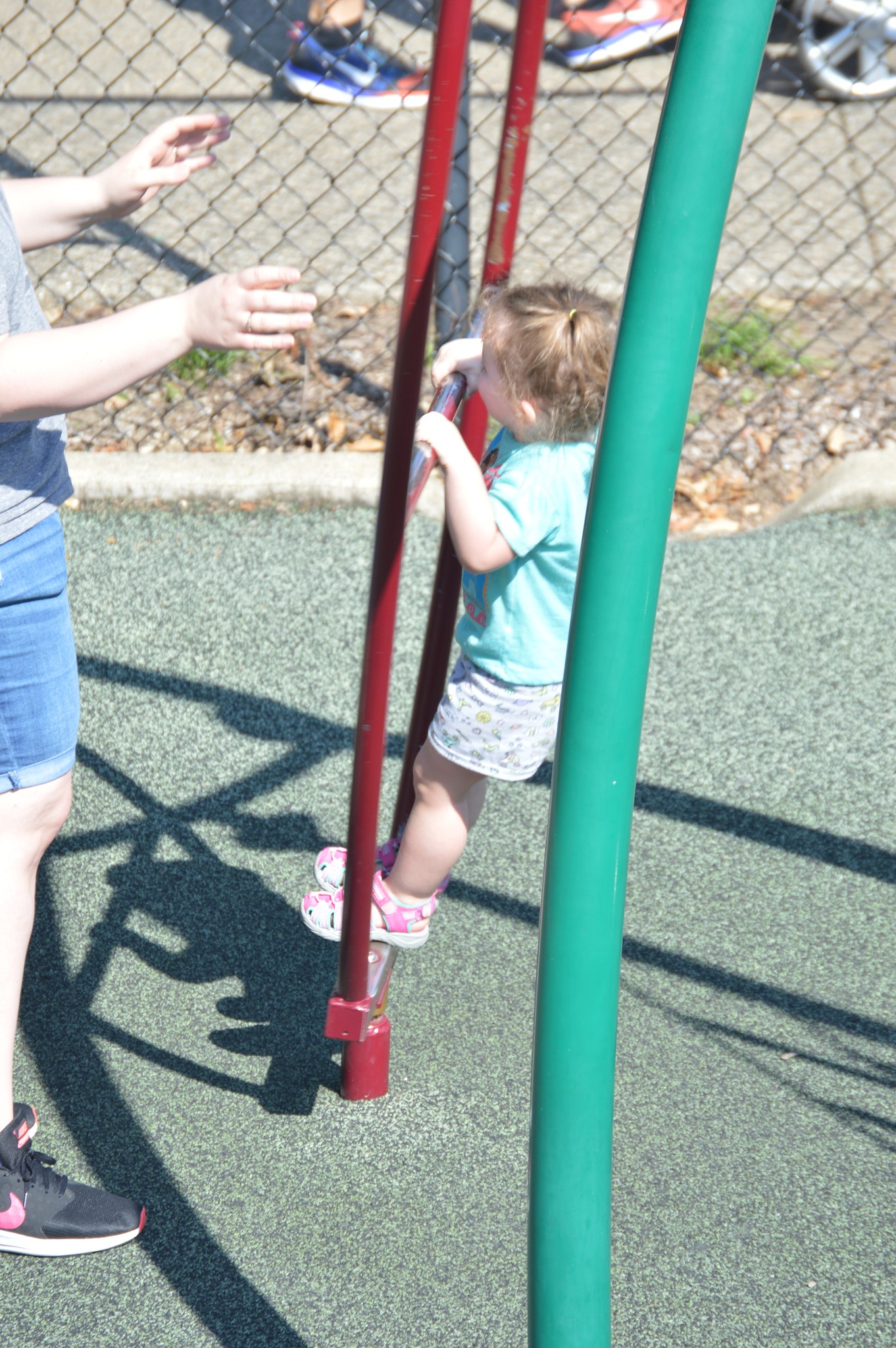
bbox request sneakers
[282,26,428,108]
[0,1124,146,1257]
[566,0,685,69]
[12,1102,38,1148]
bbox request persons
[281,1,696,113]
[299,282,630,952]
[0,113,321,1259]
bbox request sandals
[314,839,448,907]
[299,870,439,948]
